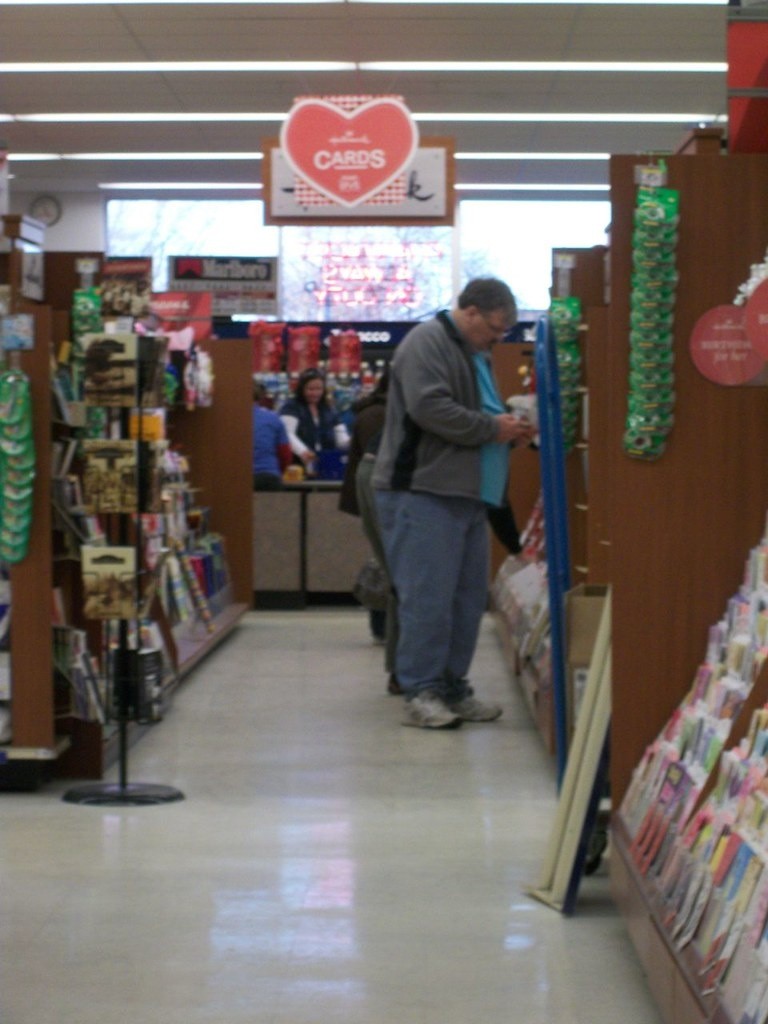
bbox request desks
[255,480,372,608]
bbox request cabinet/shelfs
[0,212,255,792]
[492,126,767,1024]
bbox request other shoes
[388,673,404,695]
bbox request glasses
[488,322,511,337]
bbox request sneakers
[400,691,463,729]
[448,697,503,722]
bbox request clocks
[29,196,60,227]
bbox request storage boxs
[564,582,609,759]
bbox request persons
[250,368,354,487]
[338,276,540,730]
